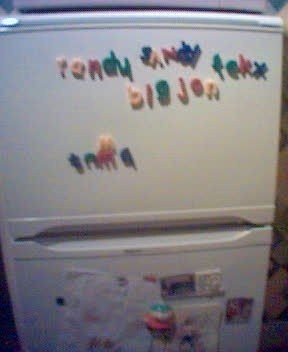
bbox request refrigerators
[1,3,286,351]
